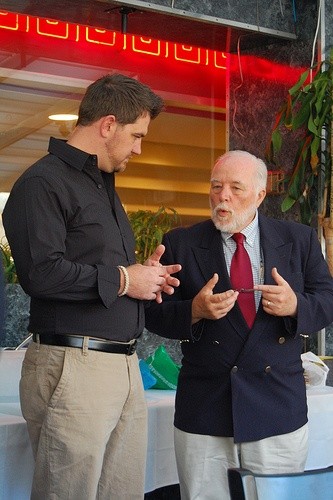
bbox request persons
[1,72,182,499]
[141,148,333,500]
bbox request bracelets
[117,265,129,297]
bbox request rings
[267,300,271,307]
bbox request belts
[32,334,138,355]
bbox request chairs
[226,465,333,500]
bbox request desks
[0,346,333,500]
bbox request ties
[229,232,256,329]
